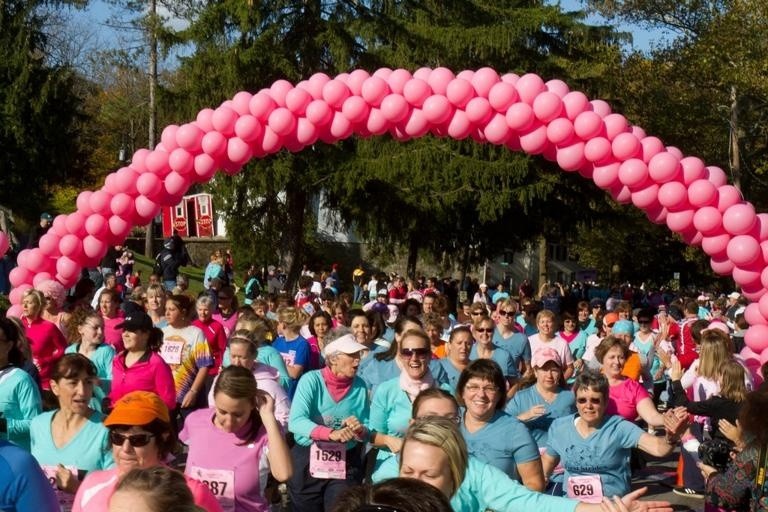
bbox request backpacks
[152,256,163,277]
[353,275,361,285]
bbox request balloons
[1,61,768,391]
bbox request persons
[1,246,767,512]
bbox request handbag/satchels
[245,283,260,300]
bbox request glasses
[476,327,493,333]
[575,397,604,404]
[109,431,166,448]
[498,310,516,316]
[400,348,429,359]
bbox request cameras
[699,439,735,467]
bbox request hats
[378,288,388,296]
[603,313,620,326]
[590,296,605,308]
[727,292,740,300]
[697,295,710,302]
[372,302,387,313]
[479,283,488,289]
[103,391,171,426]
[531,347,562,368]
[41,213,53,221]
[114,312,152,331]
[163,238,174,248]
[322,334,369,355]
